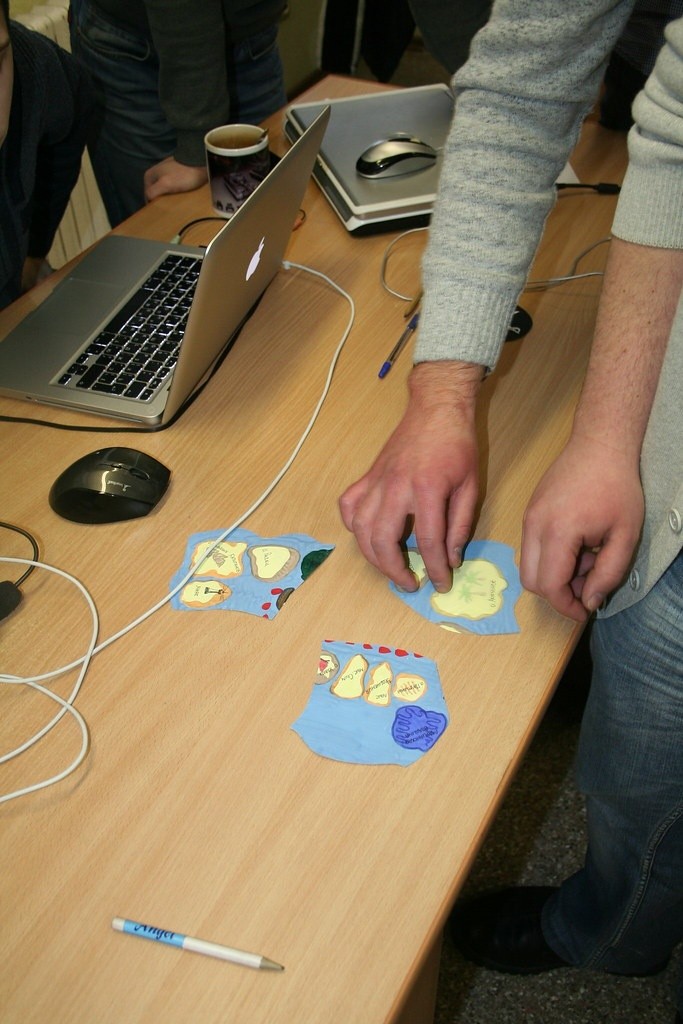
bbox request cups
[205,124,269,219]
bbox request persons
[337,0,683,593]
[456,0,683,981]
[68,0,290,230]
[1,0,97,308]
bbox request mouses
[356,136,438,179]
[49,447,171,527]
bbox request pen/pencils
[403,288,424,318]
[378,306,420,379]
[110,916,284,973]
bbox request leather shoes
[451,887,577,976]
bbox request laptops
[0,103,332,427]
[283,82,460,237]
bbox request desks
[1,76,669,1023]
